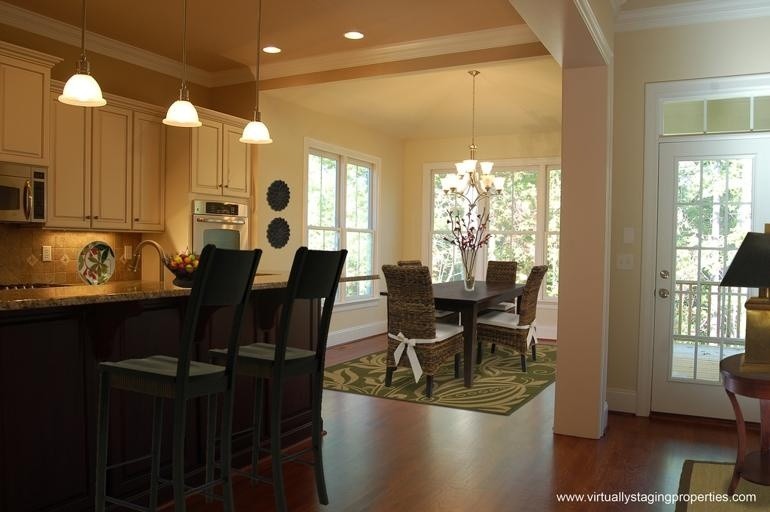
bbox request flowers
[441,206,497,274]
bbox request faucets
[128,240,165,282]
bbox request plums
[170,254,198,272]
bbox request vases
[463,264,476,291]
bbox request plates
[79,242,119,284]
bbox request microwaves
[0,159,48,224]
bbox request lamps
[439,70,504,208]
[55,0,110,111]
[158,0,203,128]
[721,220,770,373]
[236,0,275,149]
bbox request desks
[721,355,769,497]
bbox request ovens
[192,215,250,252]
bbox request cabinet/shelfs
[128,93,167,235]
[42,78,133,233]
[141,101,256,280]
[0,38,66,167]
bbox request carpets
[321,338,558,416]
[676,456,770,512]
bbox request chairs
[472,264,550,371]
[92,244,267,511]
[397,258,461,328]
[204,243,350,506]
[484,258,518,316]
[380,258,461,397]
